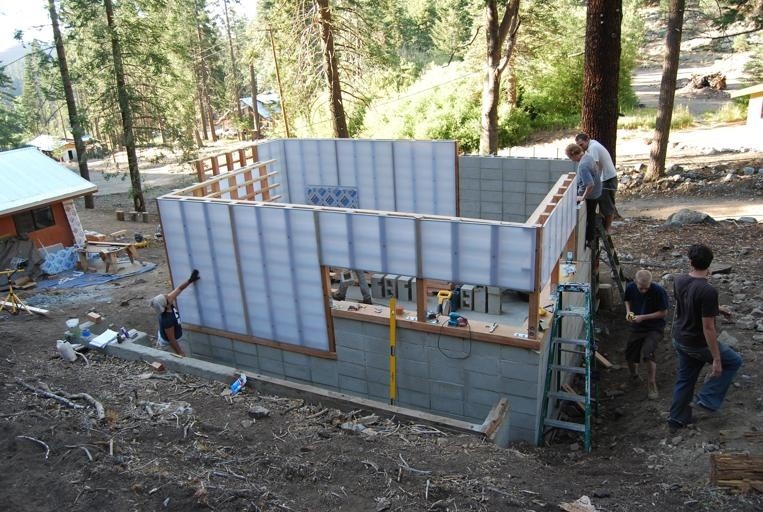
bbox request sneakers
[331,290,371,304]
[620,375,658,400]
[688,397,722,415]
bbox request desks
[77,241,144,275]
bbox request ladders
[539,283,599,452]
[592,214,626,314]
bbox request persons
[566,144,604,243]
[665,243,744,433]
[148,269,201,357]
[575,133,618,239]
[619,269,669,400]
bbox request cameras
[17,258,29,270]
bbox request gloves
[189,268,200,282]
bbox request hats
[150,294,168,314]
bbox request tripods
[0,273,34,316]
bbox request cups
[66,318,81,337]
